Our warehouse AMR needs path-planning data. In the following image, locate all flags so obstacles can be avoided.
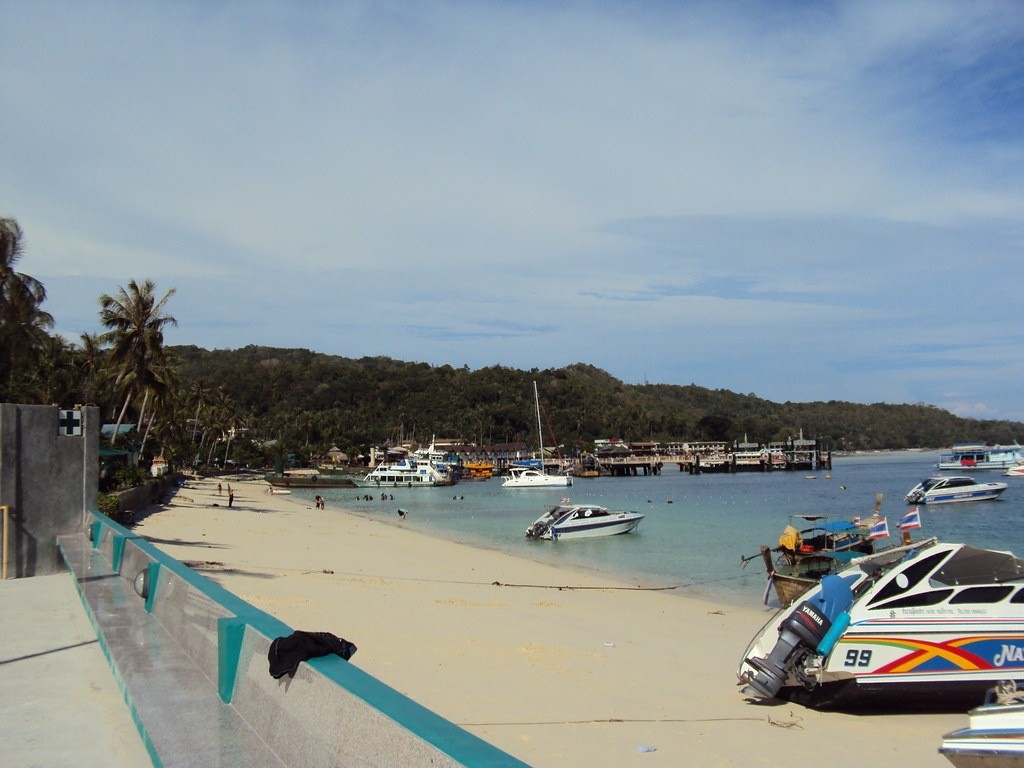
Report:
[897,508,922,530]
[867,517,890,540]
[852,516,861,527]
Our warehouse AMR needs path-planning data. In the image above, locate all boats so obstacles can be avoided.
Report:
[524,504,646,541]
[1001,465,1024,478]
[568,452,599,477]
[903,474,1009,505]
[733,504,1023,768]
[263,473,359,489]
[361,434,495,487]
[936,442,1024,469]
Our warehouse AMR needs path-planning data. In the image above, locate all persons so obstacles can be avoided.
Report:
[216,482,234,507]
[315,495,325,510]
[398,508,409,521]
[355,493,395,501]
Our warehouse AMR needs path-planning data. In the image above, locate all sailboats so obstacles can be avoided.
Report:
[500,381,574,488]
